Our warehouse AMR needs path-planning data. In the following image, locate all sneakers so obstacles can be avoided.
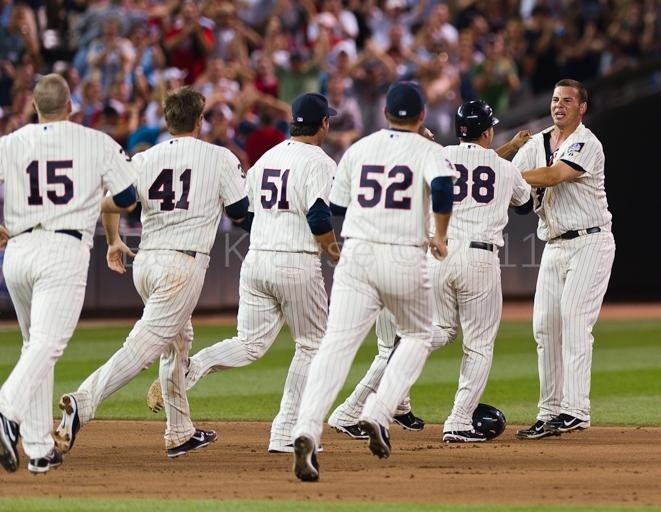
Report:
[328,421,370,439]
[146,358,192,414]
[442,428,487,443]
[268,443,324,453]
[292,433,320,482]
[516,420,553,439]
[28,447,64,476]
[543,413,591,434]
[0,412,20,474]
[52,392,79,456]
[357,417,392,460]
[392,411,426,432]
[167,428,219,459]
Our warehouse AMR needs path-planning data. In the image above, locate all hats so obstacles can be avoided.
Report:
[291,92,338,124]
[455,98,500,138]
[386,80,427,119]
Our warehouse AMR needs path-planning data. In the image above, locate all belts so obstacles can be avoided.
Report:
[175,249,196,258]
[445,239,494,252]
[21,227,82,240]
[555,227,601,240]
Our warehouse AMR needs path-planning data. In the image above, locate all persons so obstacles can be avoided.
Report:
[326,126,532,440]
[51,87,250,458]
[1,0,659,175]
[510,77,617,440]
[144,91,340,457]
[0,72,139,476]
[385,98,541,440]
[292,81,458,485]
